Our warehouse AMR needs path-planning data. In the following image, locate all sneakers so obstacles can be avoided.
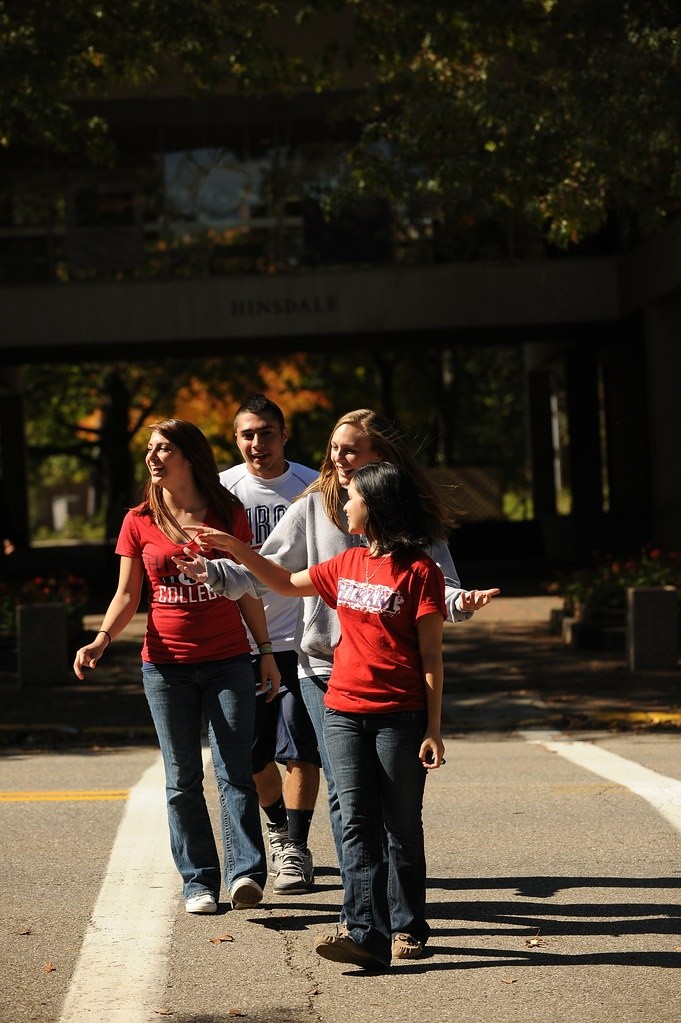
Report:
[185,893,217,913]
[230,878,263,910]
[273,839,314,894]
[391,933,423,957]
[266,822,291,876]
[314,926,389,971]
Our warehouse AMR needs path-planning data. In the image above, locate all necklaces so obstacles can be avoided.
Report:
[365,551,389,587]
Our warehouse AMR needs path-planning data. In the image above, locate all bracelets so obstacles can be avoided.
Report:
[258,641,274,655]
[97,631,111,646]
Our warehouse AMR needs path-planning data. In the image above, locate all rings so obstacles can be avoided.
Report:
[193,574,197,579]
[440,759,446,765]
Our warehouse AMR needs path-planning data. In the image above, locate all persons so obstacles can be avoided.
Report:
[73,416,281,910]
[180,460,464,969]
[171,410,500,936]
[213,395,323,889]
